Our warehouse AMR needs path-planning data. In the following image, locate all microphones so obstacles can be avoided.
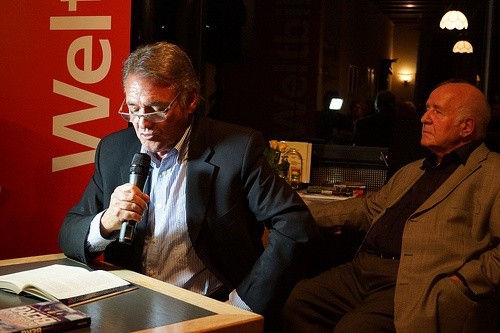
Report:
[118,153,151,245]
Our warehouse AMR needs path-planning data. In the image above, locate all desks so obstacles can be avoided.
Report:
[0,253,264,333]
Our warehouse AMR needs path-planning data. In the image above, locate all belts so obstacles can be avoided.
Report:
[360,245,400,261]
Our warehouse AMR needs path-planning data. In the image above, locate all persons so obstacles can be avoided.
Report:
[57,40,318,333]
[262,82,500,333]
[206,71,472,186]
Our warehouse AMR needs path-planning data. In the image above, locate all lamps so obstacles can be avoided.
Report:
[439,7,469,31]
[452,35,474,54]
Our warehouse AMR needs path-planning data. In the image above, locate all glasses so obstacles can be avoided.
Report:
[117,84,184,123]
